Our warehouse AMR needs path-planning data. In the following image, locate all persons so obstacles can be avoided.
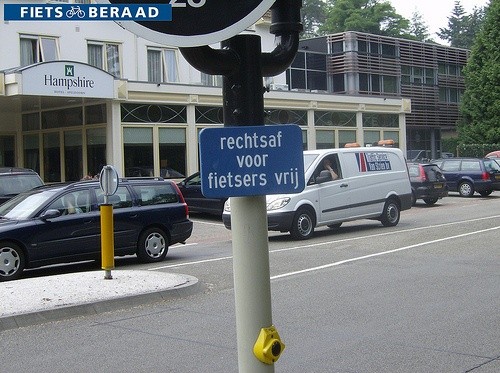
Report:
[324,158,338,180]
[60,194,83,216]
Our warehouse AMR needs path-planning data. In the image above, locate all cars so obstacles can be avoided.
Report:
[177,171,228,218]
[406,146,455,162]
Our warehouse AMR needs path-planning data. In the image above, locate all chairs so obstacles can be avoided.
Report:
[77,193,86,213]
[49,199,62,209]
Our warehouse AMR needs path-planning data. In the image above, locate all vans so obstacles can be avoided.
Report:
[0,176,193,281]
[222,146,413,240]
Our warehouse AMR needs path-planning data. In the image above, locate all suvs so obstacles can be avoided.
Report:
[0,167,46,207]
[430,157,500,197]
[407,162,447,206]
